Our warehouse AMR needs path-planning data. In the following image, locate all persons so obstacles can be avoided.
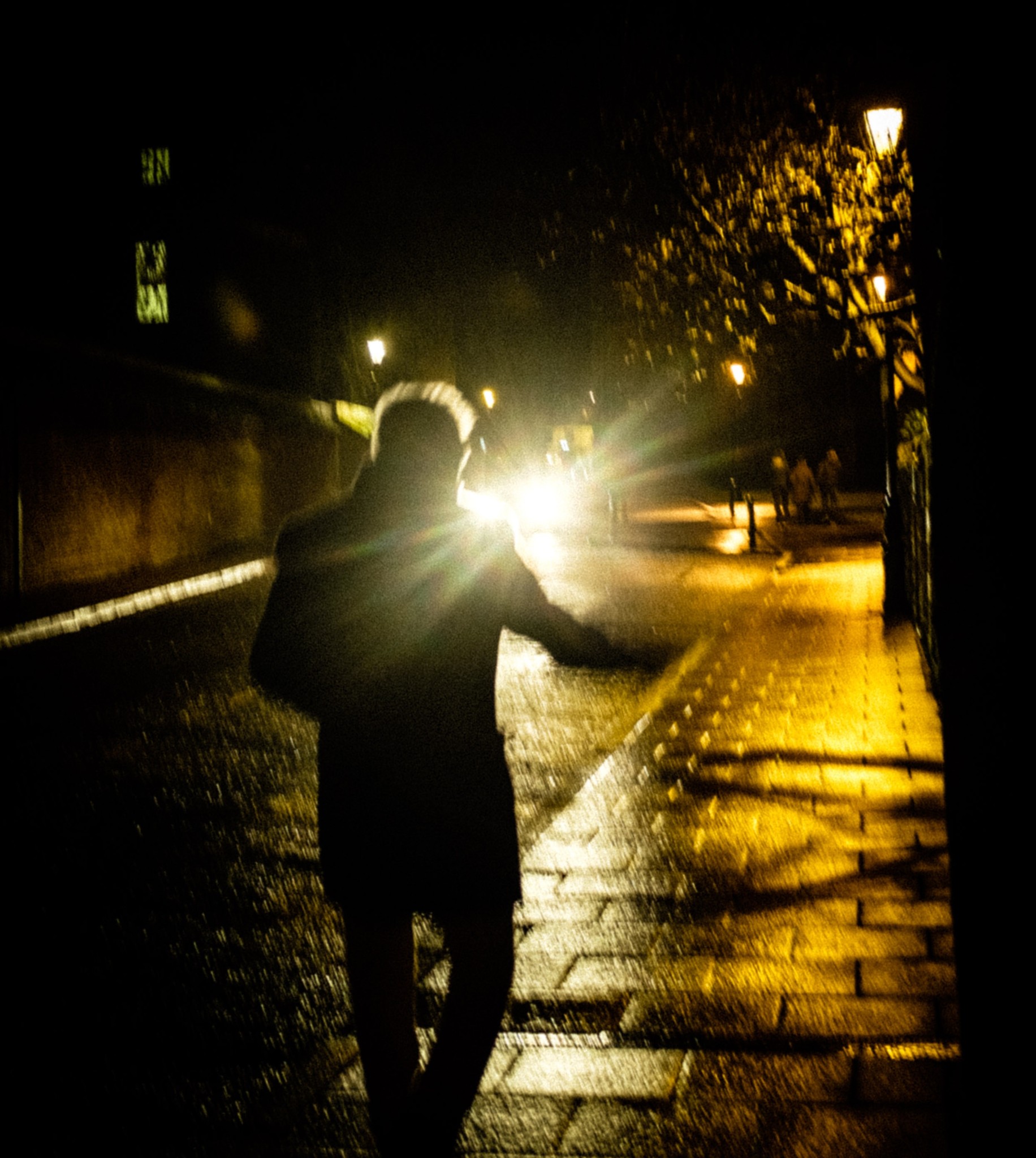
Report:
[249,388,616,1158]
[817,449,842,524]
[789,455,818,522]
[769,449,792,521]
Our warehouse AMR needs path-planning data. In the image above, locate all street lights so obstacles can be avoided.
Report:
[860,104,908,639]
[728,360,758,555]
